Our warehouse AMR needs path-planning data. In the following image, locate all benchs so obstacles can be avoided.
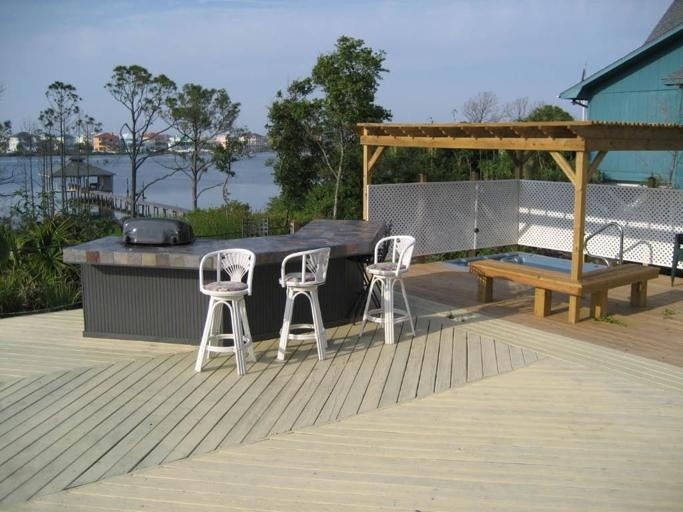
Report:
[468,258,661,324]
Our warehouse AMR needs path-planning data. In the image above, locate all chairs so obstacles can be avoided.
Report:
[358,234,416,345]
[193,247,257,376]
[343,219,391,325]
[275,247,332,361]
